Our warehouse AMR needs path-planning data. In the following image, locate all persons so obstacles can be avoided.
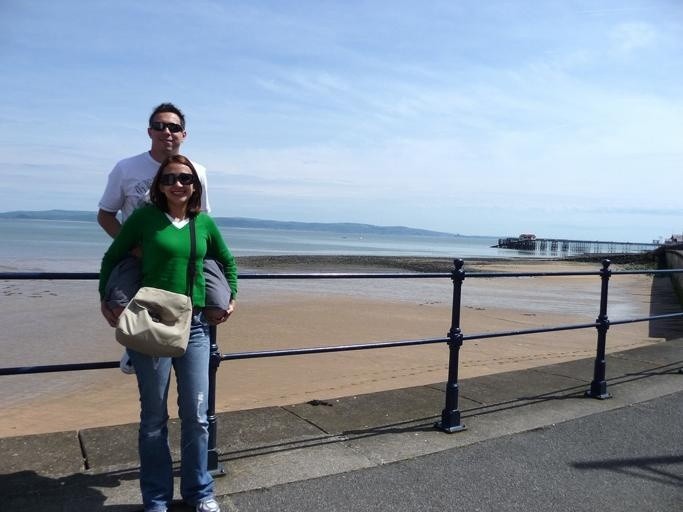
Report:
[98,152,240,511]
[97,102,232,373]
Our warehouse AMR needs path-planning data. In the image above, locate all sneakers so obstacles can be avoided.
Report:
[183,496,221,512]
[120,351,136,374]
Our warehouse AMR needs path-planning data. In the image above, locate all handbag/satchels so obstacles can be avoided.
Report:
[115,287,193,358]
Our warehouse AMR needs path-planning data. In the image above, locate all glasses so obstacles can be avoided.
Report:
[150,122,183,132]
[158,174,195,185]
